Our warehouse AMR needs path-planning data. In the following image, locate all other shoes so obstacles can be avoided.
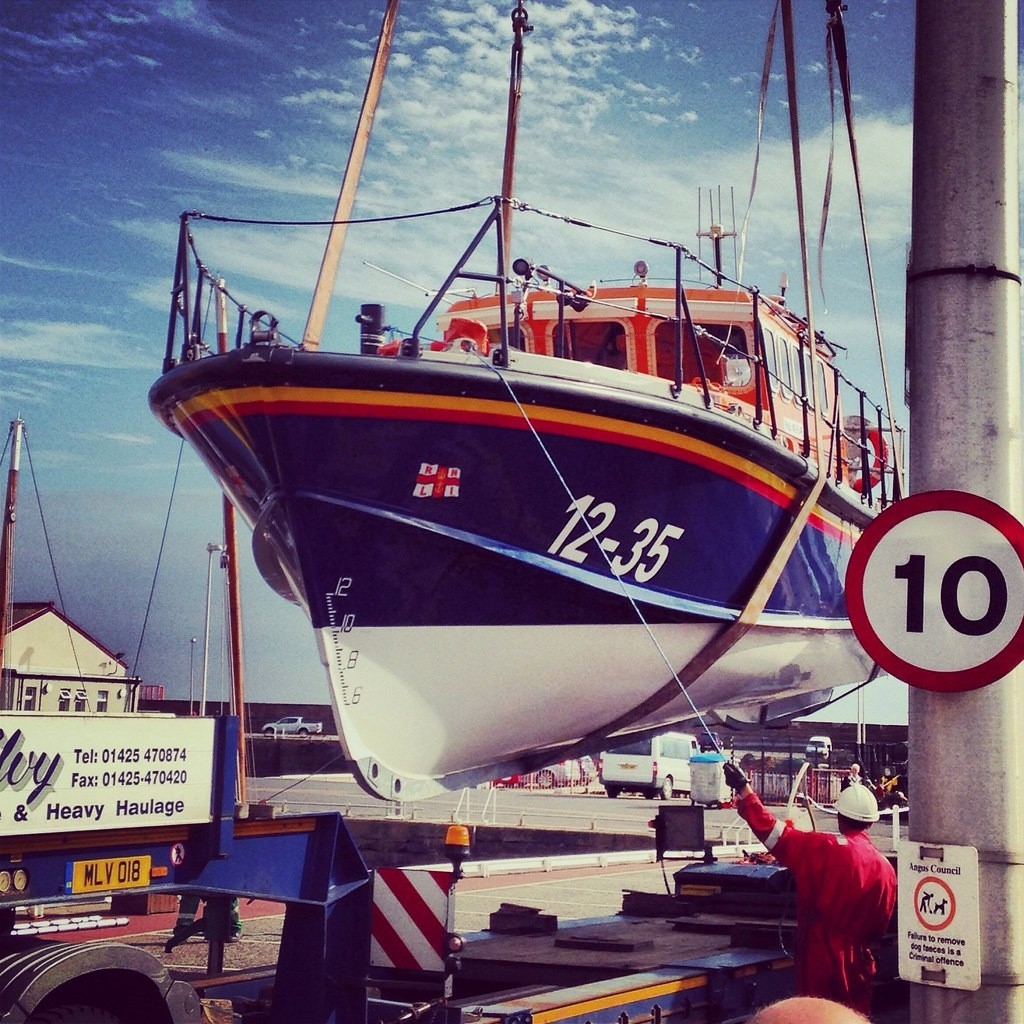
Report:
[226,930,242,942]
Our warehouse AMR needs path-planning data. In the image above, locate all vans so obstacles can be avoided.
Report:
[597,730,701,800]
[805,735,832,760]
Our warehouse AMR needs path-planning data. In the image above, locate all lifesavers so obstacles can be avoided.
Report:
[848,431,890,492]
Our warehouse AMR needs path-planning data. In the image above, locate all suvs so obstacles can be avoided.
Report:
[698,731,725,755]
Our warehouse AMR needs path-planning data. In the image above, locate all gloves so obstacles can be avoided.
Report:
[723,764,752,793]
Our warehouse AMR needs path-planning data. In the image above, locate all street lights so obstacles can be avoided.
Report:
[198,542,224,716]
[189,637,198,716]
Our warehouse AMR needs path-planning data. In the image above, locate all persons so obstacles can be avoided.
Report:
[722,762,897,1019]
[840,764,864,792]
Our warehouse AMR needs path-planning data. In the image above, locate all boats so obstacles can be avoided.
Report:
[140,0,908,806]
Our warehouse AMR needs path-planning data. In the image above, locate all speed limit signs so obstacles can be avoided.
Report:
[843,490,1024,695]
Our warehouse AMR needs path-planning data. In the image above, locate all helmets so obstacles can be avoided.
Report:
[834,783,879,822]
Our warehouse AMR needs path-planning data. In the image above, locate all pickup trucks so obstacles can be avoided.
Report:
[261,716,323,738]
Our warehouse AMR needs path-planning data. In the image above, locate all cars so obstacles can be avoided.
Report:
[492,755,598,790]
